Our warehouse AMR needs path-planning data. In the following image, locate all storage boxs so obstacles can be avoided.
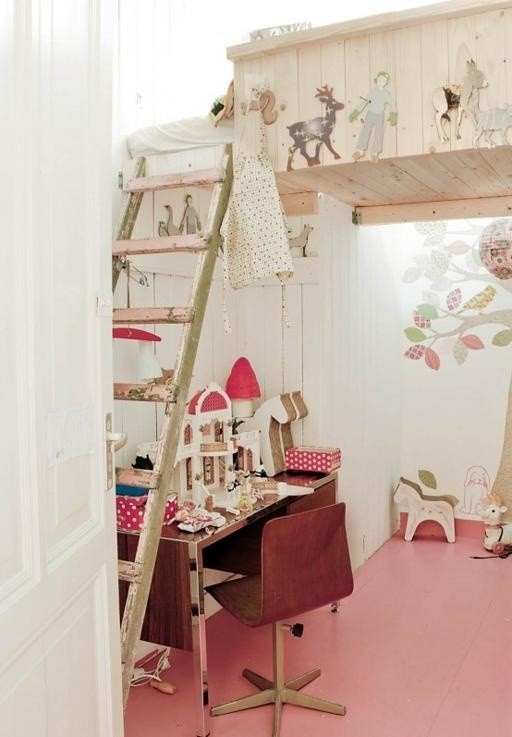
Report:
[117,492,177,530]
[286,445,343,475]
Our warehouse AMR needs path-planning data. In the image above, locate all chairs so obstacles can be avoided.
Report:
[206,503,353,735]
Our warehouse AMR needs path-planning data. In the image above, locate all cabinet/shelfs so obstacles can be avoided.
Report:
[118,453,339,655]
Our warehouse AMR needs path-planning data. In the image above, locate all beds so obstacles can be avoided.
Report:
[124,98,252,159]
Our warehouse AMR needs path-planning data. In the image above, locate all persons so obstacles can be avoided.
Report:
[178,194,204,237]
[346,71,398,164]
